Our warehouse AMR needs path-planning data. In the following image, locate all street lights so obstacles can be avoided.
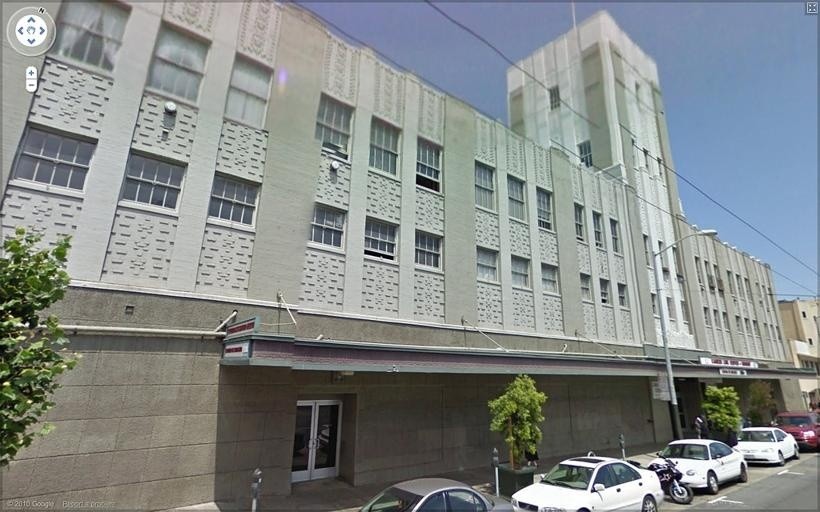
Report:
[650,227,718,440]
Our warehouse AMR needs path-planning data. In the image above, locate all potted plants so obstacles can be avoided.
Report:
[487,371,550,499]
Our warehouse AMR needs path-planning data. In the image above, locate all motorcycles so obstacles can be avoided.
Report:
[625,452,695,505]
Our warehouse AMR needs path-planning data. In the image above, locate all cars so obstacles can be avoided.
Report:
[646,438,748,495]
[510,455,664,512]
[767,410,820,453]
[358,477,514,511]
[734,426,800,469]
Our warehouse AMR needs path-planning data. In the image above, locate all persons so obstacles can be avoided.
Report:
[743,416,752,429]
[690,411,704,440]
[524,448,540,468]
[809,398,820,414]
